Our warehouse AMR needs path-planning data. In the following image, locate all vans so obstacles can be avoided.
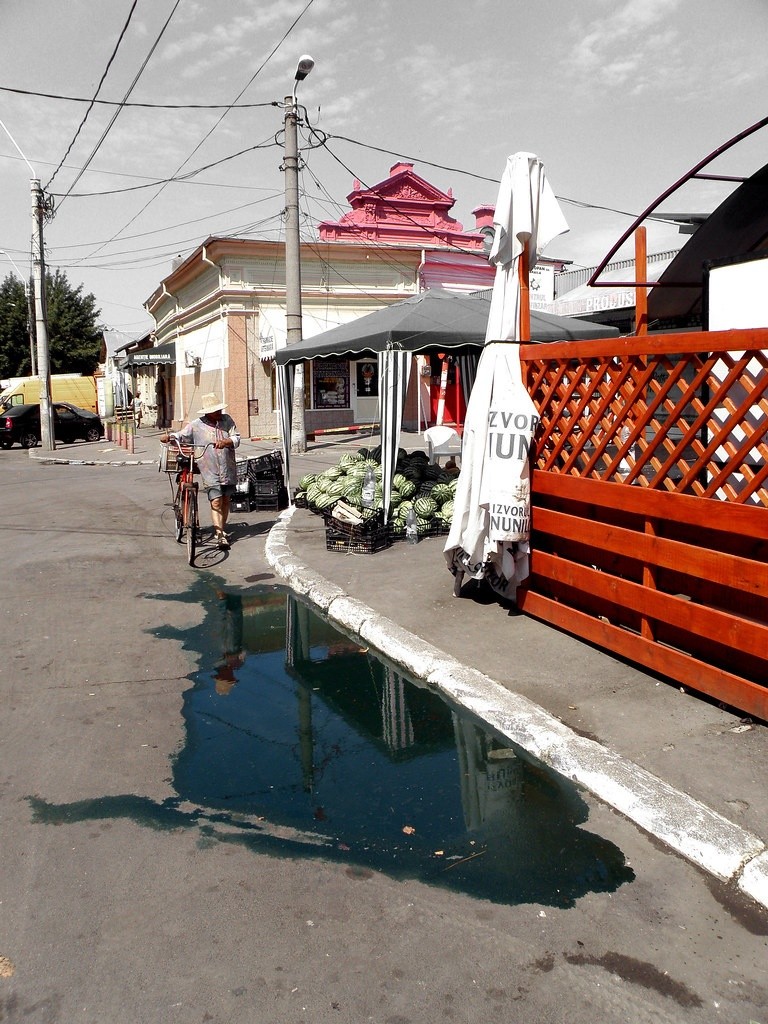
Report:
[0,377,99,416]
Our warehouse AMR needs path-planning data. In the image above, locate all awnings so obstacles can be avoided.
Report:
[117,342,176,371]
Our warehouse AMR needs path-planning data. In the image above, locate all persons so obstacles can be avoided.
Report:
[161,393,240,548]
[130,391,142,428]
[210,591,247,694]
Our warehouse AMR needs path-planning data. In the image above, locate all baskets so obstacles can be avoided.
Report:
[229,450,287,512]
[323,496,385,555]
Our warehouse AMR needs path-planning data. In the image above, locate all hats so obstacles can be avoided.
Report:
[196,392,228,414]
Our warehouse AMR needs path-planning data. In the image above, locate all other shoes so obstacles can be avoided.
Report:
[214,530,231,540]
[215,539,229,549]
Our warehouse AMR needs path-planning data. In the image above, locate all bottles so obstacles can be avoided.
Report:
[362,469,376,508]
[407,507,417,547]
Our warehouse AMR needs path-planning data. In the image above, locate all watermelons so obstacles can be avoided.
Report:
[295,444,462,535]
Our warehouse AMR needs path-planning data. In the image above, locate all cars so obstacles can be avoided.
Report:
[0,402,106,450]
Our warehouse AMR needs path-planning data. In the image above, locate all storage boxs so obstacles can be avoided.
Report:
[230,493,256,513]
[322,497,392,554]
[255,466,283,479]
[250,452,283,472]
[235,476,254,493]
[256,488,288,512]
[254,476,284,496]
[162,446,201,474]
[236,460,254,481]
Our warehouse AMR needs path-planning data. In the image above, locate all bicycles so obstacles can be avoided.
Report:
[171,436,216,567]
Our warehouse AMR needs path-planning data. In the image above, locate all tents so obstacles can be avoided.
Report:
[275,288,620,526]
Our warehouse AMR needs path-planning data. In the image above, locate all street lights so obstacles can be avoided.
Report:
[284,52,317,455]
[0,249,37,376]
